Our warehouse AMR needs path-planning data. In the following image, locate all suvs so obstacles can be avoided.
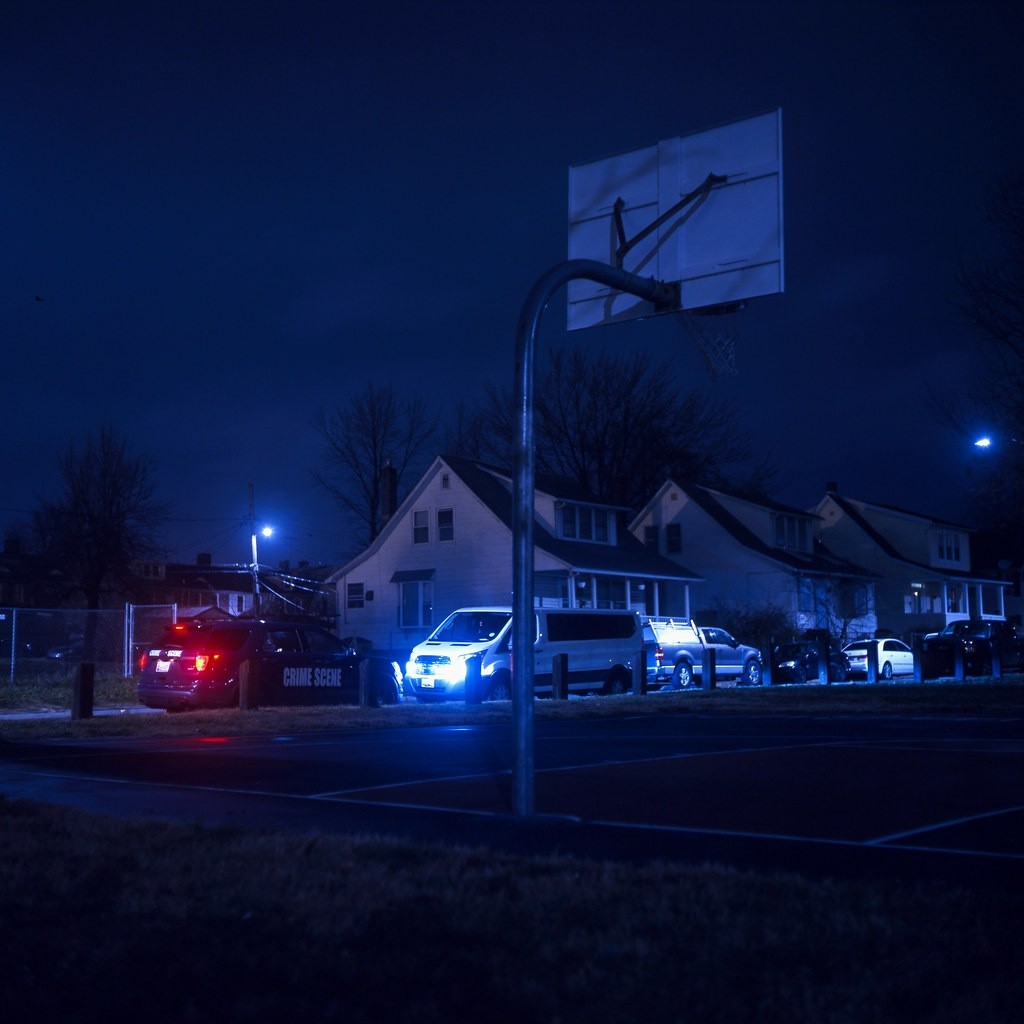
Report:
[136,618,359,709]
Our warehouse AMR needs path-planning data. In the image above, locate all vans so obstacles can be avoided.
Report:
[403,606,648,703]
[640,615,762,689]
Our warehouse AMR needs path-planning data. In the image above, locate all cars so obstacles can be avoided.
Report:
[338,636,398,707]
[773,641,849,685]
[840,639,916,680]
[97,647,121,662]
[916,616,1023,676]
[24,641,47,657]
[45,648,72,662]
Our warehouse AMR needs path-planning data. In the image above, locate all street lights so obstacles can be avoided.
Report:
[252,526,272,611]
